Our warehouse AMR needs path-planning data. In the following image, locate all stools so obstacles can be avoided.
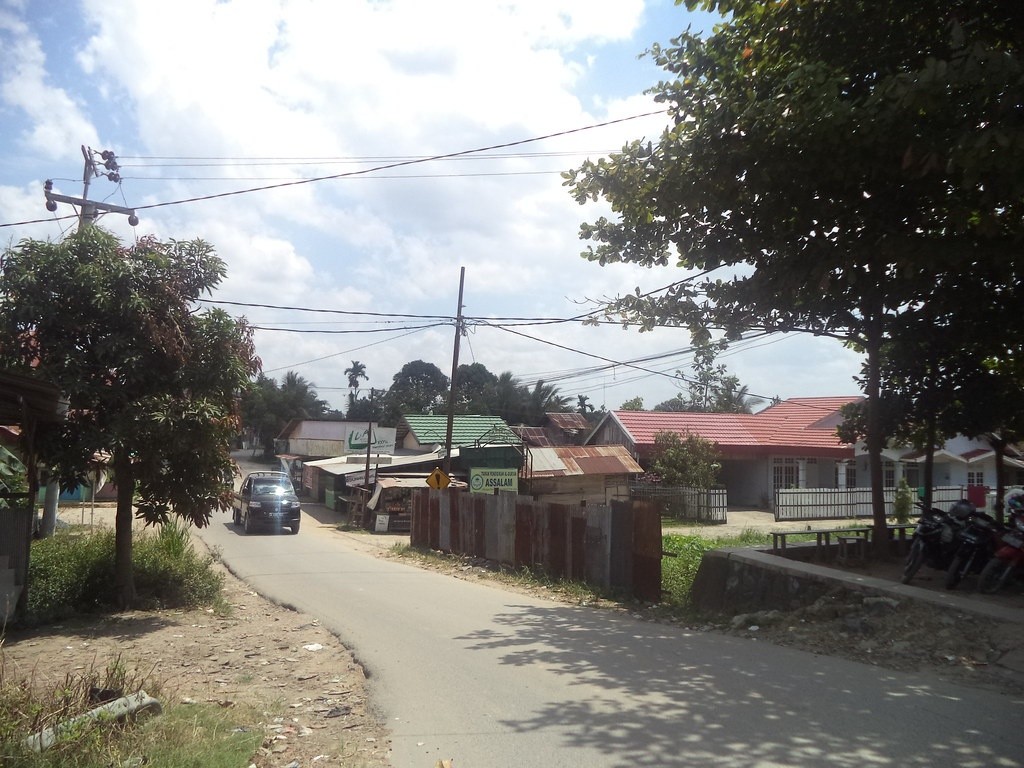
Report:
[836,536,867,570]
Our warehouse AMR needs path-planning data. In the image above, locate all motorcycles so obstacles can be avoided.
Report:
[903,496,977,585]
[944,504,1003,591]
[974,488,1024,595]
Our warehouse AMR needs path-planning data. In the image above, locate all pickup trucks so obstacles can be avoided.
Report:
[230,470,302,535]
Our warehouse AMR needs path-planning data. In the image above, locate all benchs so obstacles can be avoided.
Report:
[867,524,917,556]
[769,527,872,561]
